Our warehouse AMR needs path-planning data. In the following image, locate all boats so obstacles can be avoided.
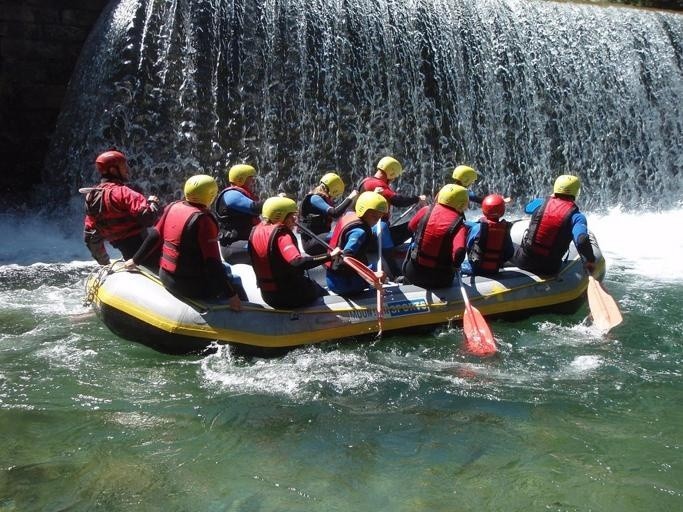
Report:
[83,227,606,356]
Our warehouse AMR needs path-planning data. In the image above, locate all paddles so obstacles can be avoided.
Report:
[295,222,379,287]
[455,269,496,356]
[376,219,385,329]
[572,237,623,330]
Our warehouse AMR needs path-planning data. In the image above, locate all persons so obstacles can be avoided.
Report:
[359,155,426,277]
[453,167,483,228]
[124,175,249,311]
[388,184,470,290]
[214,164,286,265]
[299,172,360,256]
[508,175,596,276]
[78,150,162,275]
[324,191,389,297]
[248,196,344,310]
[461,193,515,279]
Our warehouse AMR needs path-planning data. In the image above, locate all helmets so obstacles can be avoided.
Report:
[452,165,478,186]
[320,173,345,197]
[261,197,300,225]
[354,190,389,218]
[184,174,219,209]
[439,183,469,213]
[229,164,257,187]
[482,194,505,220]
[553,175,581,202]
[377,156,403,180]
[96,152,129,176]
